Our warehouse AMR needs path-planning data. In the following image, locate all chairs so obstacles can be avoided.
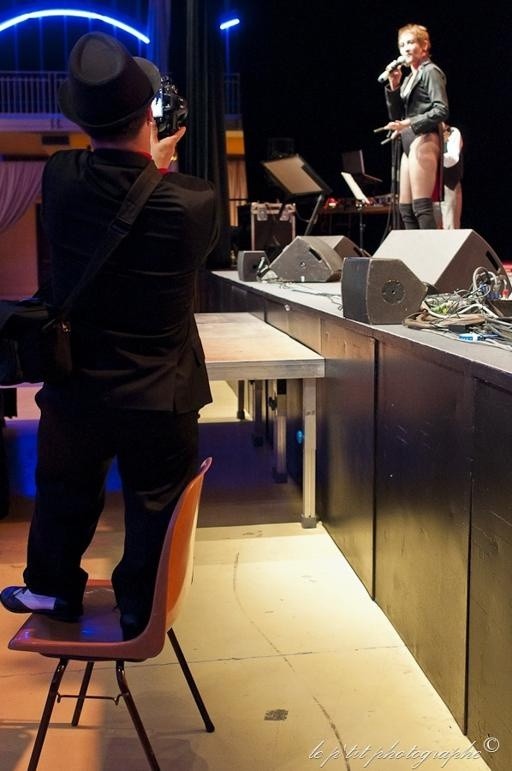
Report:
[8,457,214,771]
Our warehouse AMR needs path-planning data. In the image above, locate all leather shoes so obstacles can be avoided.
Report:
[118,604,148,631]
[0,585,85,622]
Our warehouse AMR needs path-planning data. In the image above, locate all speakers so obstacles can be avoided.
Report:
[237,202,296,264]
[270,236,343,282]
[371,229,512,300]
[318,236,359,261]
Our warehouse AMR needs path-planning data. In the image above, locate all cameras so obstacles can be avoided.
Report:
[151,74,190,140]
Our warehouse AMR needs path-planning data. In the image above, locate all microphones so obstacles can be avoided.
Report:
[378,56,405,84]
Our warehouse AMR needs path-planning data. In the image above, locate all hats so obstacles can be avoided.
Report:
[58,32,162,132]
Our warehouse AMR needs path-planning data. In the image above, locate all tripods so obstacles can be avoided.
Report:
[357,203,370,257]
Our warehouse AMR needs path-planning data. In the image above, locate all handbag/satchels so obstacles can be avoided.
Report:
[1,297,81,388]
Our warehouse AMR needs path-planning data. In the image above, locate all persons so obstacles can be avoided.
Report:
[385,24,450,229]
[0,29,218,640]
[434,122,464,232]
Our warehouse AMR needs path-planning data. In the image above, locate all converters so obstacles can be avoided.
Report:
[449,323,468,334]
